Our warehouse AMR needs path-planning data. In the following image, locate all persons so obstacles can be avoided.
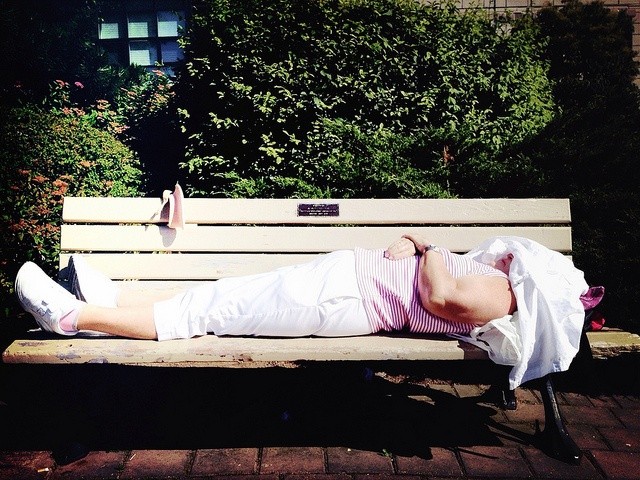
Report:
[13,234,519,342]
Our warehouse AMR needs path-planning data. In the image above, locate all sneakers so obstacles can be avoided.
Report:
[68,255,119,335]
[14,261,79,336]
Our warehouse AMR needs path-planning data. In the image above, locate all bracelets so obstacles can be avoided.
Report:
[422,244,442,255]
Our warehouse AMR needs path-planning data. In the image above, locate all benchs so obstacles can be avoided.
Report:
[3,196,639,462]
